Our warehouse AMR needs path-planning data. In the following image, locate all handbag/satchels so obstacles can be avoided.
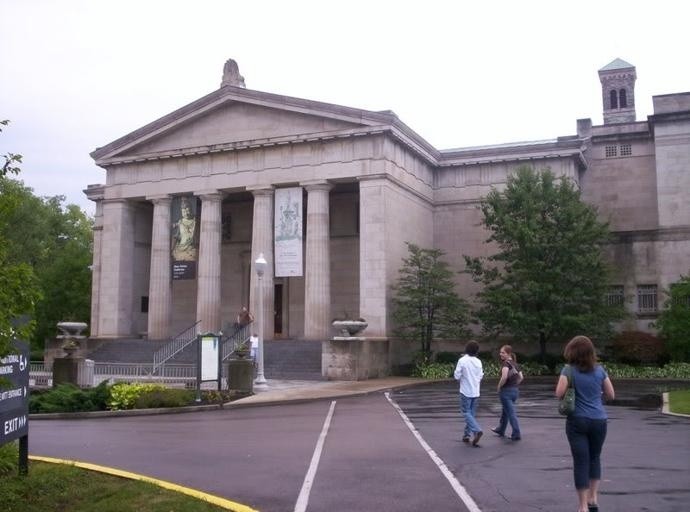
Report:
[506,361,518,384]
[558,366,576,416]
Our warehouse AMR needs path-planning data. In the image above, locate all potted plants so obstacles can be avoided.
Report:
[331,311,371,336]
[55,311,87,336]
[231,344,251,358]
[60,342,81,357]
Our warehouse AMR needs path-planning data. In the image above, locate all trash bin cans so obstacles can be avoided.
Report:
[85,360,94,387]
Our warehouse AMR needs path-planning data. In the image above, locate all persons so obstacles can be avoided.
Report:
[237,306,254,339]
[491,345,525,441]
[555,336,615,512]
[453,340,484,446]
[171,197,198,261]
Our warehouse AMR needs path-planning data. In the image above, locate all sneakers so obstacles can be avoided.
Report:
[472,431,483,445]
[463,438,469,442]
[587,503,599,512]
[491,427,504,436]
[508,436,521,440]
